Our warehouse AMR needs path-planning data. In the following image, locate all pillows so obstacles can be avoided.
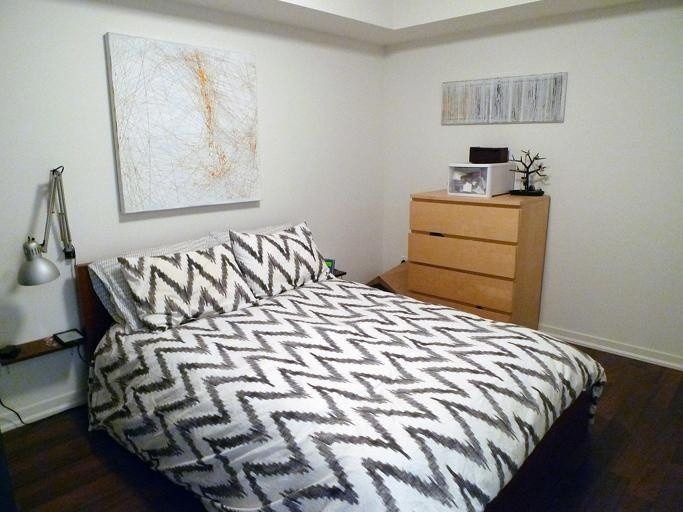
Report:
[87,222,339,329]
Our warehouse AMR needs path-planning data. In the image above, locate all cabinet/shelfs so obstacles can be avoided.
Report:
[405,191,550,331]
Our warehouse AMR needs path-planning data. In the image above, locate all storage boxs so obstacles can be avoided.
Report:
[446,161,515,198]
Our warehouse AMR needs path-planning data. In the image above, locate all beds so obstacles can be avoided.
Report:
[73,221,607,459]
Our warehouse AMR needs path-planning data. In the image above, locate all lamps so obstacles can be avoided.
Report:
[17,166,75,286]
